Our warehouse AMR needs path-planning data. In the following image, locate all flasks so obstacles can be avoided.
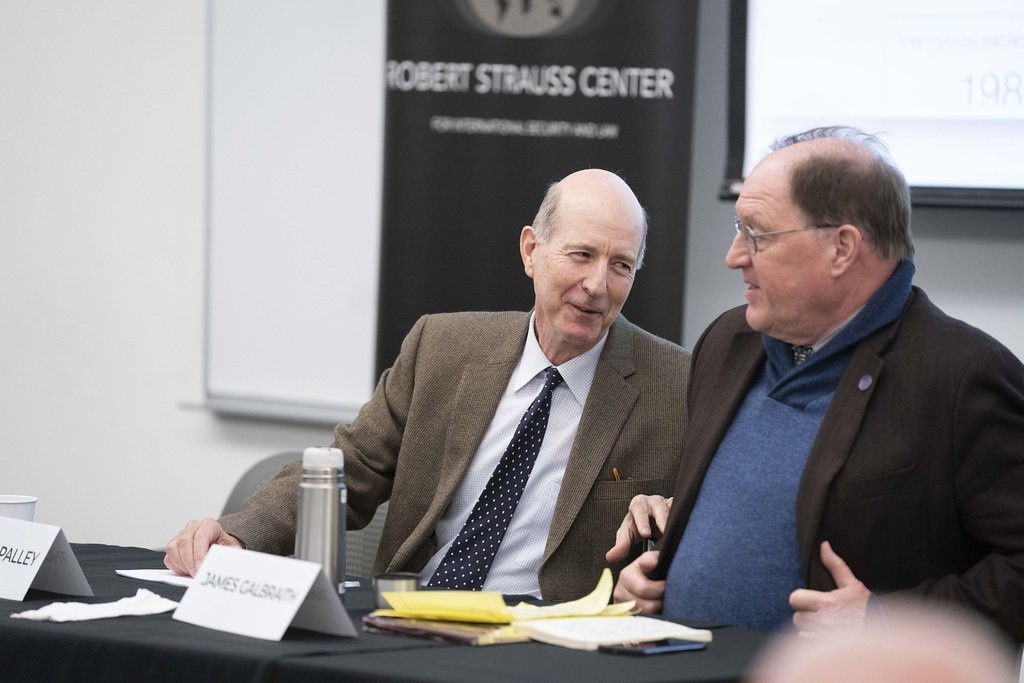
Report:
[293,447,347,609]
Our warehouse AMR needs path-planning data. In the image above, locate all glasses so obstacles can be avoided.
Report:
[733,216,851,256]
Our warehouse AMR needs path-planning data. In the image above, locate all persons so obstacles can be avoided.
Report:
[613,126,1024,648]
[163,170,692,608]
[754,597,1008,683]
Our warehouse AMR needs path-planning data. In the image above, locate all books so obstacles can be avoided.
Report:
[363,568,712,650]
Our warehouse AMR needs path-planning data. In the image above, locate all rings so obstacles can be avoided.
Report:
[666,497,673,505]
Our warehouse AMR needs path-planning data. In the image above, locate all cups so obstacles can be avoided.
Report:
[0,495,38,523]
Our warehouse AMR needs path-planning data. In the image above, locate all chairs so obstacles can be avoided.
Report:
[222,451,391,576]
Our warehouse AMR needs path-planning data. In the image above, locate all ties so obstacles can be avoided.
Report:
[425,366,564,589]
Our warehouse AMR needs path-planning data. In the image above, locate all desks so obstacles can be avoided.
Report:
[0,538,772,683]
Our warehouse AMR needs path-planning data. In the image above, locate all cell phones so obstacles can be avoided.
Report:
[597,636,706,655]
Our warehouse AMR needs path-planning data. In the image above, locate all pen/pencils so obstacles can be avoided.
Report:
[613,467,621,481]
